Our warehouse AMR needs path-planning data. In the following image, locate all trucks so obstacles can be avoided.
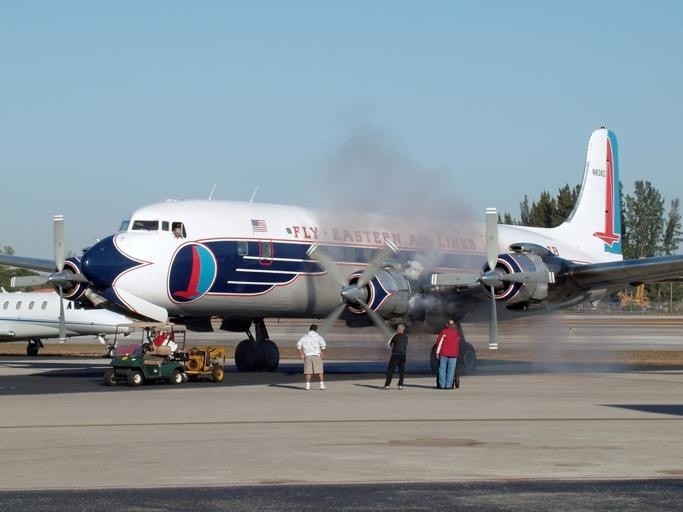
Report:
[108,320,227,386]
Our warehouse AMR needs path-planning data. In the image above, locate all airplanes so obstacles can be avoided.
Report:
[0,284,135,355]
[0,124,683,378]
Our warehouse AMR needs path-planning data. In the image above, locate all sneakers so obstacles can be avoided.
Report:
[397,386,401,390]
[319,385,325,390]
[305,386,310,390]
[381,386,389,390]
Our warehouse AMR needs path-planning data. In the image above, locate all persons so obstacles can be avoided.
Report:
[296,324,327,391]
[436,319,461,389]
[381,324,409,390]
[174,227,182,239]
[152,328,178,358]
